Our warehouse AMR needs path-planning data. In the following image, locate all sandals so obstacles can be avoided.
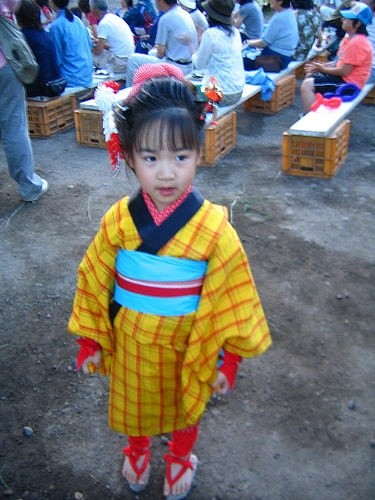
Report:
[123,450,150,492]
[161,455,198,499]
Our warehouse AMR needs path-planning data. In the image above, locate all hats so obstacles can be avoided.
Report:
[178,0,198,11]
[202,0,235,25]
[340,2,372,25]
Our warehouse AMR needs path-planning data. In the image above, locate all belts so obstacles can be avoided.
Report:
[167,57,193,65]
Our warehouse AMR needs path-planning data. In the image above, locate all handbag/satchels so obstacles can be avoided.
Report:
[0,17,39,85]
[45,77,66,97]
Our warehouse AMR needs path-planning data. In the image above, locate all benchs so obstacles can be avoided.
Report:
[289,82,375,137]
[312,37,338,56]
[28,72,126,102]
[205,50,319,128]
[79,86,132,111]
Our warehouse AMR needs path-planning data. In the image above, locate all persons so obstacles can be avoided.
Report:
[125,0,199,88]
[115,0,165,55]
[67,0,135,74]
[14,0,67,98]
[67,63,273,500]
[177,0,209,46]
[0,0,48,201]
[298,4,373,118]
[34,0,93,88]
[191,0,246,108]
[242,0,299,74]
[231,0,264,44]
[290,0,375,84]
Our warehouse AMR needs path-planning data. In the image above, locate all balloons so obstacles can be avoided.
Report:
[310,92,342,111]
[324,83,360,102]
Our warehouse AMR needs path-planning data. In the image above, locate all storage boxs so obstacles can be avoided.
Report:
[292,49,330,81]
[26,94,78,140]
[241,74,296,117]
[74,108,108,149]
[196,111,238,168]
[282,118,351,179]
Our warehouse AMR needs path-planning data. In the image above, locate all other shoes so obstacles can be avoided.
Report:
[25,179,47,203]
